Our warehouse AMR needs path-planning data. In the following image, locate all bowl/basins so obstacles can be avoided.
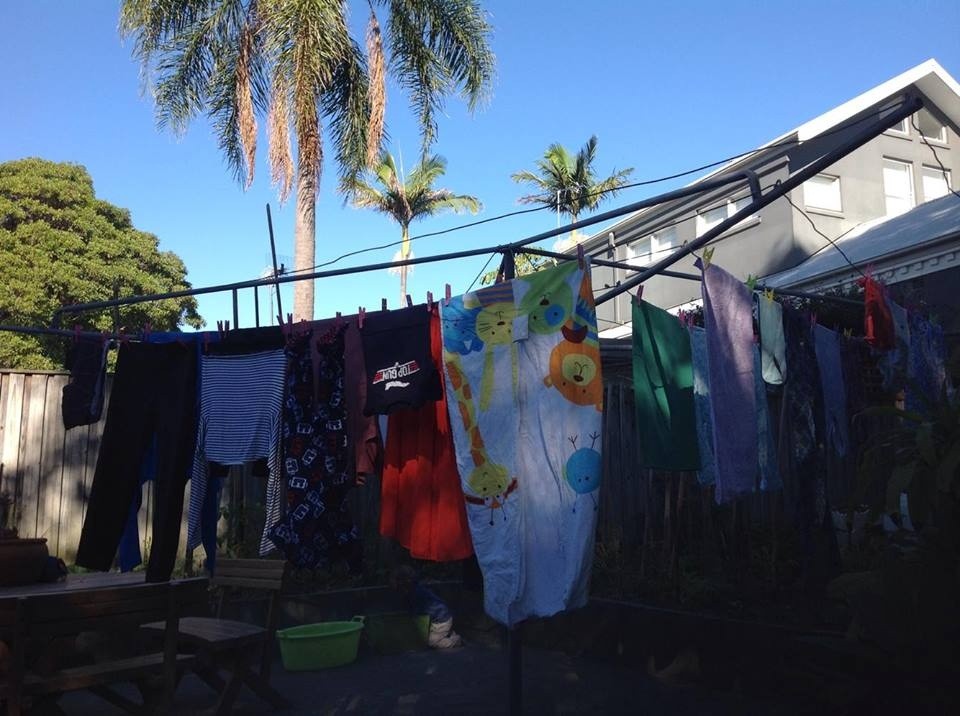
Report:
[275,616,366,670]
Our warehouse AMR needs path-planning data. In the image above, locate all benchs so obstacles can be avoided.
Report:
[0,559,289,716]
